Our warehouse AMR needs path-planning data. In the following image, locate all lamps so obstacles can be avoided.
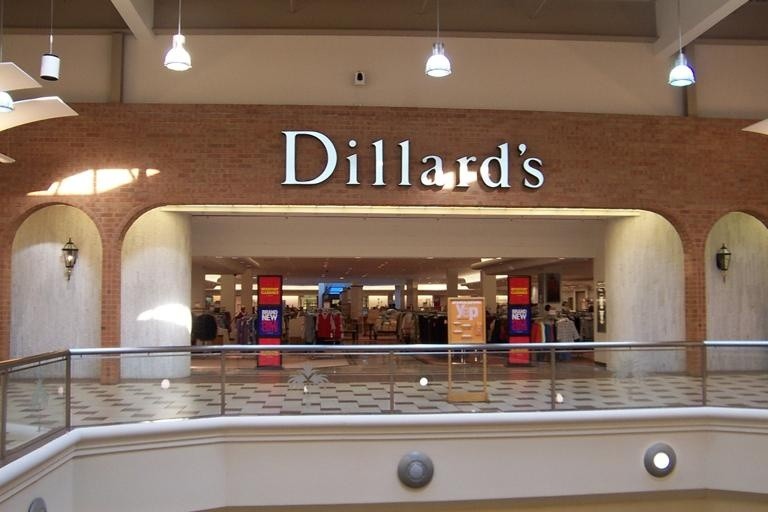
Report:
[0,91,14,112]
[716,241,732,277]
[668,0,696,88]
[165,0,194,72]
[39,0,60,82]
[425,0,451,77]
[62,238,78,280]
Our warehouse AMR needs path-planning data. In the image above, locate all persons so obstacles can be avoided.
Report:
[584,298,594,312]
[485,308,498,342]
[235,304,248,319]
[560,301,571,317]
[545,303,561,316]
[365,306,380,341]
